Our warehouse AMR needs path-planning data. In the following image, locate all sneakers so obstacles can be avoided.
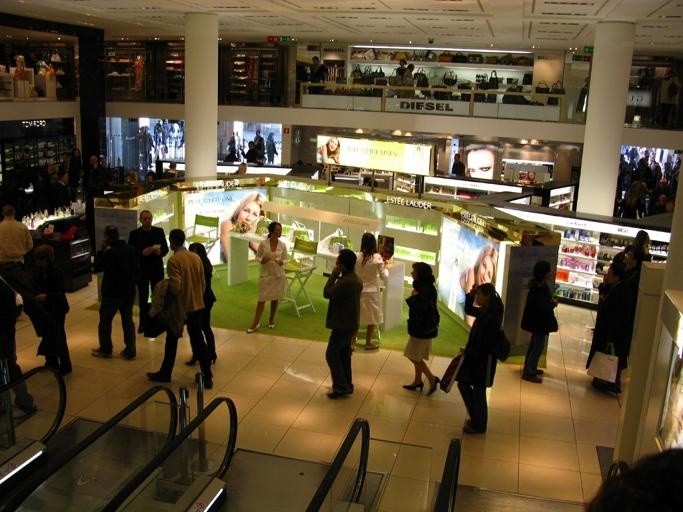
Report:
[92,348,136,360]
[522,369,543,383]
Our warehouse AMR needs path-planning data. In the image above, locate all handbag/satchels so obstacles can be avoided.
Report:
[336,65,428,87]
[536,80,564,105]
[432,70,533,103]
[440,355,463,393]
[352,48,534,66]
[587,351,621,381]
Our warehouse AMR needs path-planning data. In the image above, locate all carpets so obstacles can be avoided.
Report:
[83,260,550,372]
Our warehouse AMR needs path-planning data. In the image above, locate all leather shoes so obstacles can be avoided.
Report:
[248,319,274,333]
[147,372,171,382]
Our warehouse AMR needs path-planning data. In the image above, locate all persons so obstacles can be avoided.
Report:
[89,226,139,359]
[184,243,216,366]
[396,59,405,76]
[451,154,466,179]
[146,229,214,389]
[220,192,267,263]
[308,56,329,93]
[43,148,106,204]
[84,170,157,251]
[613,149,680,224]
[137,120,185,179]
[228,129,278,174]
[469,146,506,185]
[520,260,561,384]
[247,222,288,333]
[128,211,169,334]
[583,230,653,397]
[317,139,339,168]
[308,231,504,434]
[2,205,74,412]
[405,65,414,77]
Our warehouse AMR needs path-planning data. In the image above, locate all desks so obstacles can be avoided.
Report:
[225,229,406,334]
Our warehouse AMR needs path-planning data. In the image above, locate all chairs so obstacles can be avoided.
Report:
[278,237,318,318]
[179,214,218,276]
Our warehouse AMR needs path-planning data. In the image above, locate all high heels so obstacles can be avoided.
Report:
[186,353,217,365]
[403,377,440,395]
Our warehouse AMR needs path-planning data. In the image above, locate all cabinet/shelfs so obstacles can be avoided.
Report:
[345,43,533,90]
[551,212,671,311]
[0,133,77,184]
[105,41,296,110]
